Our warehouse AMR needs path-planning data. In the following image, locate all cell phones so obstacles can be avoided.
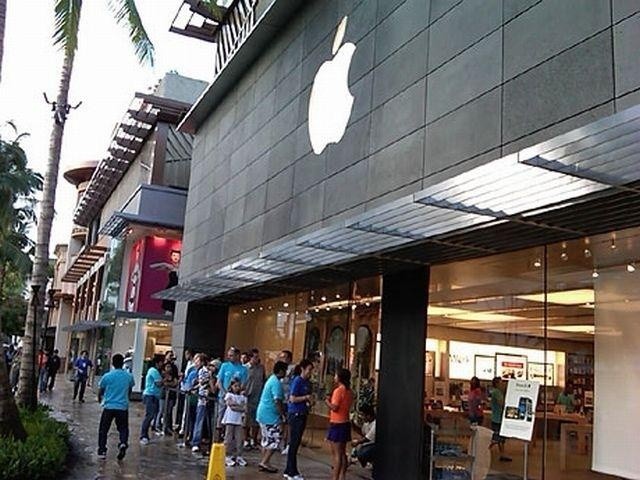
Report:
[519,398,526,419]
[526,398,532,421]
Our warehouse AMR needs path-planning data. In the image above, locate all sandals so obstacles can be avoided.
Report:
[259,461,278,473]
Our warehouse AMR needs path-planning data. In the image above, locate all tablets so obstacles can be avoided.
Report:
[505,406,520,419]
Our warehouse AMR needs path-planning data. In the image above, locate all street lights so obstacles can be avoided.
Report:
[29,282,60,411]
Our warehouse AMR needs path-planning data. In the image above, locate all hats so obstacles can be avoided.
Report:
[207,359,222,369]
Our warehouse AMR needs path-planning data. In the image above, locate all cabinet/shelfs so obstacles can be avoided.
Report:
[555,347,594,407]
[424,423,477,480]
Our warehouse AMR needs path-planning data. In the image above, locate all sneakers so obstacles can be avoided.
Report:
[283,471,305,480]
[116,444,128,460]
[498,454,513,462]
[138,428,199,452]
[233,456,248,466]
[244,439,259,451]
[225,455,236,466]
[95,449,107,459]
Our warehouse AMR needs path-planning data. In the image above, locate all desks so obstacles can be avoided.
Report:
[559,419,595,474]
[531,411,589,454]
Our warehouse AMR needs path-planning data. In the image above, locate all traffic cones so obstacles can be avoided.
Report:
[205,440,226,479]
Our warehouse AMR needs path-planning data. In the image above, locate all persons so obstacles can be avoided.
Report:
[149,249,180,316]
[488,376,513,464]
[469,375,486,427]
[557,383,575,414]
